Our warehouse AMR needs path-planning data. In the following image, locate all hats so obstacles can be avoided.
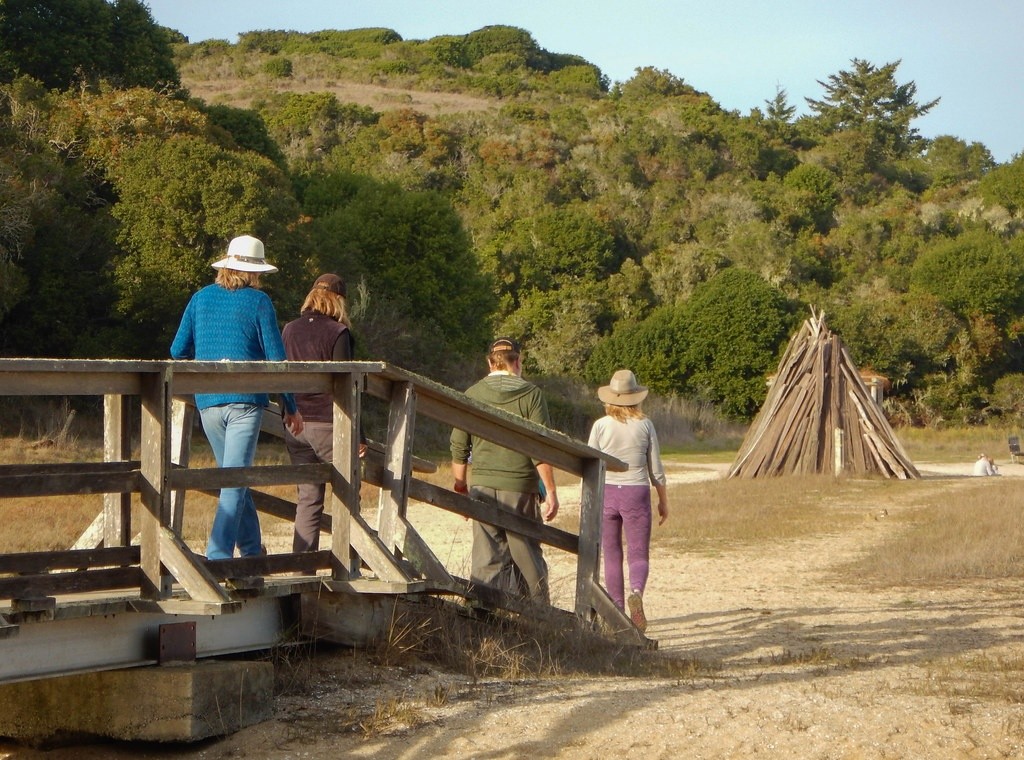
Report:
[598,370,649,407]
[312,273,347,300]
[211,235,279,275]
[488,337,521,355]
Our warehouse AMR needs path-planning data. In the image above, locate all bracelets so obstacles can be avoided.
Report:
[454,484,467,492]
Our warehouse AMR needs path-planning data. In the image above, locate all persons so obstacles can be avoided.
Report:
[281,273,368,576]
[170,235,304,560]
[588,370,669,634]
[974,453,998,476]
[450,337,559,606]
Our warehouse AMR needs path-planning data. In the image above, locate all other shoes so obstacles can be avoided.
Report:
[626,591,648,634]
